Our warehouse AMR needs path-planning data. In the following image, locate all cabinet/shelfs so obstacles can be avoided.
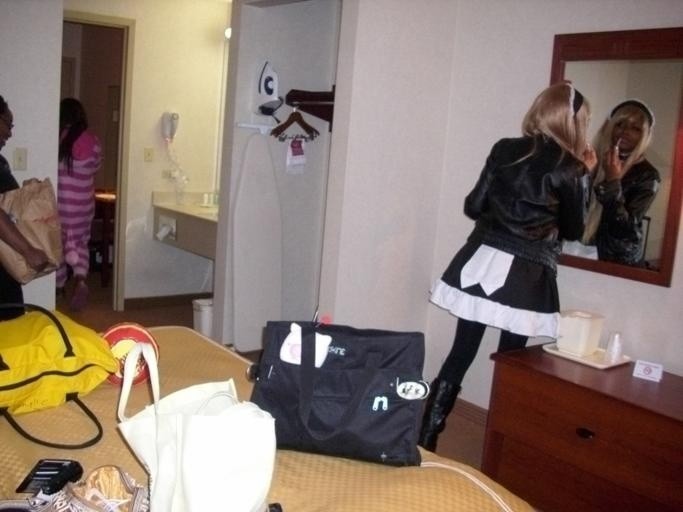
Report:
[480,340,683,510]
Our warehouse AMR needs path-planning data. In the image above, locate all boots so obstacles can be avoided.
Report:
[418,378,461,453]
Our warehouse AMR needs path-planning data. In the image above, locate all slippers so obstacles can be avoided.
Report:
[70,282,88,310]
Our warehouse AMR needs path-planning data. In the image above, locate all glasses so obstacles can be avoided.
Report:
[1,119,17,132]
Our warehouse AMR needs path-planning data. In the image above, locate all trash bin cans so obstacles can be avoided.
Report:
[192,298,212,339]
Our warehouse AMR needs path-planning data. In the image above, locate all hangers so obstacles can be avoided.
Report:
[269,102,319,142]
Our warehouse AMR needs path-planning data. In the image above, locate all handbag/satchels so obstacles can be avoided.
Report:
[0,309,116,414]
[0,177,64,285]
[117,378,277,512]
[247,319,425,468]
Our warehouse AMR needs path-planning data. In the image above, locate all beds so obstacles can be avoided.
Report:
[1,325,534,511]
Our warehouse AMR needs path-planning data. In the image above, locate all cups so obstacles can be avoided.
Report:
[606,330,623,365]
[200,193,216,206]
[555,308,605,358]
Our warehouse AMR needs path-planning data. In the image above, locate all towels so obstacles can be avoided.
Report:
[286,137,307,175]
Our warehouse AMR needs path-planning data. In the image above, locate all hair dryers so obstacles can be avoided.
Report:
[162,112,178,144]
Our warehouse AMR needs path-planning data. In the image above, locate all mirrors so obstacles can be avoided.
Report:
[549,28,683,289]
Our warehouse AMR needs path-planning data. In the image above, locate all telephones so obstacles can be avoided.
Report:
[163,112,179,141]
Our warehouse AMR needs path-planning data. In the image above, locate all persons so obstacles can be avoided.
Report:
[56,98,104,311]
[581,99,662,267]
[0,95,48,320]
[417,79,598,453]
